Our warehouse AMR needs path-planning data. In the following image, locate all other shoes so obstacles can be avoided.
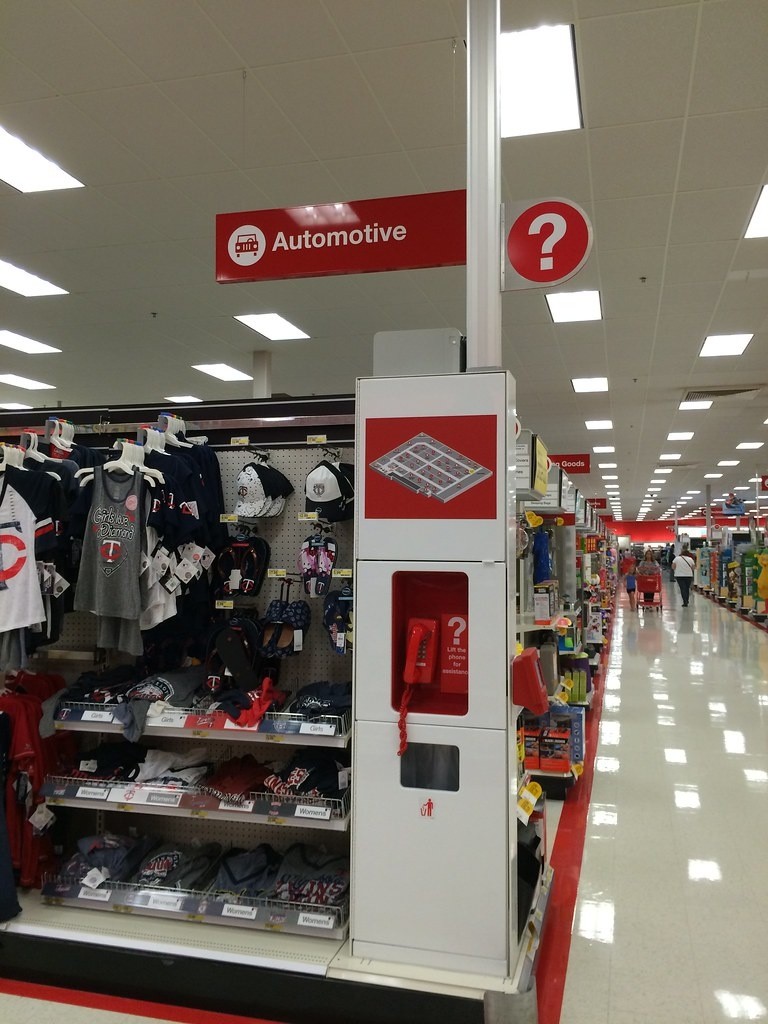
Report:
[633,608,636,612]
[646,607,649,609]
[670,580,676,582]
[631,609,633,611]
[687,600,689,604]
[650,606,652,608]
[256,600,312,659]
[682,604,688,607]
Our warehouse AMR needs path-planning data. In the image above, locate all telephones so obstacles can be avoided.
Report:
[402,617,439,684]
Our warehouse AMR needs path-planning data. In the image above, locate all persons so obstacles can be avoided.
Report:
[619,548,630,560]
[671,551,696,607]
[622,564,637,612]
[638,549,662,613]
[669,547,677,582]
[679,546,697,591]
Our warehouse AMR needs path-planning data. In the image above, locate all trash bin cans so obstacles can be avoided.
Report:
[349,720,518,978]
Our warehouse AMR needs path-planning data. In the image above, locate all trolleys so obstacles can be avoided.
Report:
[635,568,664,613]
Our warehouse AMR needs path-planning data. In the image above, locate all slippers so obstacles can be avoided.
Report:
[217,536,241,596]
[346,600,353,648]
[322,590,345,651]
[298,536,321,594]
[315,536,338,596]
[240,537,271,597]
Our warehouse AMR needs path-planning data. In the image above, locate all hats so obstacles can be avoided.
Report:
[305,460,354,522]
[234,462,295,517]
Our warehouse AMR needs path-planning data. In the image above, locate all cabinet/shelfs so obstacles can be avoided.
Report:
[515,430,617,801]
[40,680,350,940]
